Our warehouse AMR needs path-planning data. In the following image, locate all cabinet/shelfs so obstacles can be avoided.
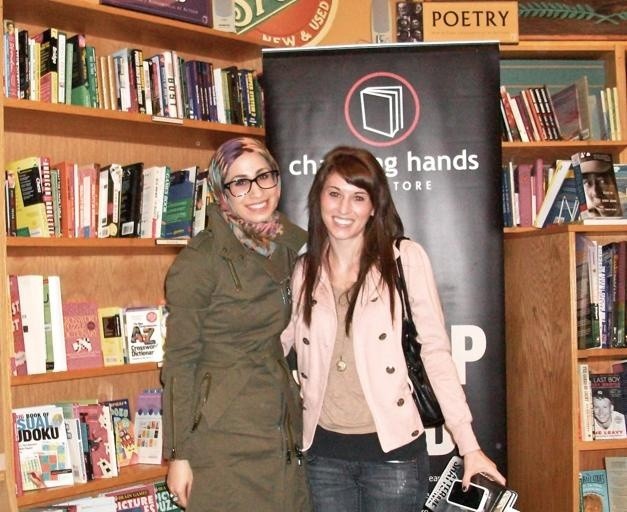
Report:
[493,34,627,512]
[1,2,278,512]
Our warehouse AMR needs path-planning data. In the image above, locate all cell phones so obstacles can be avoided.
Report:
[446,480,490,512]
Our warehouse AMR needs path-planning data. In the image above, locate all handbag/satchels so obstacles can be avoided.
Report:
[401,320,444,430]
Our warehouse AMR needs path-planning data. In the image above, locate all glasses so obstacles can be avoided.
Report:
[221,168,279,198]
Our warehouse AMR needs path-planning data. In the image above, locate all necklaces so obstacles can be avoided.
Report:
[336,331,346,372]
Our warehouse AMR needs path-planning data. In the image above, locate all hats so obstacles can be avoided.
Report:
[578,152,611,175]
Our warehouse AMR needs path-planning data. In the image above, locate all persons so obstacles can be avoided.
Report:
[281,147,519,511]
[157,134,313,511]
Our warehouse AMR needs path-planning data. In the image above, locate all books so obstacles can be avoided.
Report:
[13,388,164,494]
[3,19,261,128]
[574,232,627,350]
[5,156,213,238]
[6,274,168,374]
[503,487,519,512]
[502,77,620,142]
[578,362,627,441]
[31,480,184,512]
[579,457,627,512]
[425,454,512,512]
[501,152,627,228]
